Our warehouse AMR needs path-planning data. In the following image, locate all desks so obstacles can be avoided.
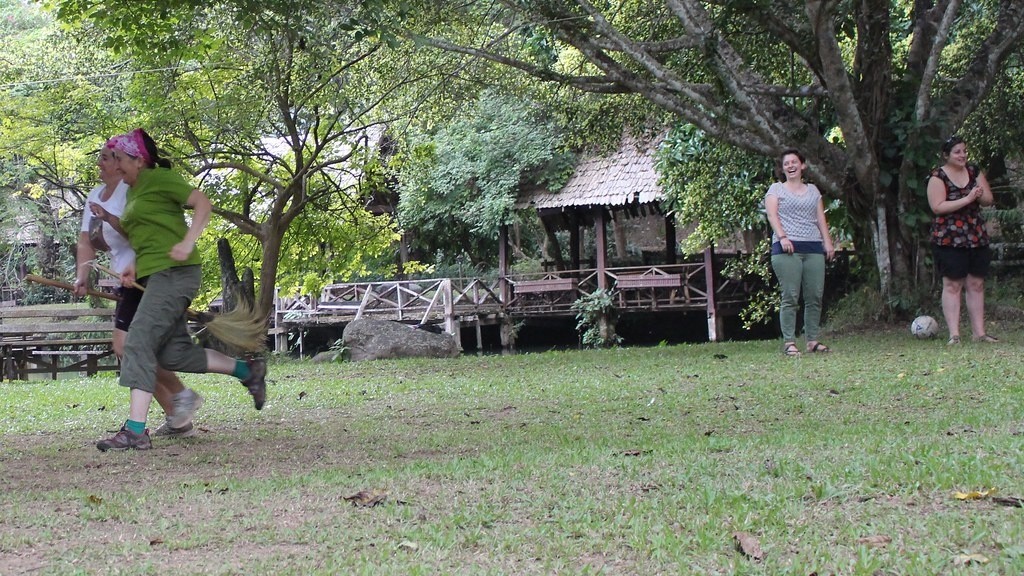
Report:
[616,271,657,307]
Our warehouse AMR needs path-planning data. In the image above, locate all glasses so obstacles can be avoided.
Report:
[943,135,963,152]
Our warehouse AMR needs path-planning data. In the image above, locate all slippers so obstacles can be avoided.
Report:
[806,341,832,353]
[783,344,802,355]
[947,336,962,347]
[971,333,1000,343]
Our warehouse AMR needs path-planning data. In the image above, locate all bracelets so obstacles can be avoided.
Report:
[778,235,787,241]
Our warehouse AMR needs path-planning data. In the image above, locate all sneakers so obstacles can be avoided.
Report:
[152,421,196,440]
[96,419,153,453]
[238,356,267,410]
[169,388,204,429]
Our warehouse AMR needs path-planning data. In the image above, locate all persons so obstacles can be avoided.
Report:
[926,136,1001,347]
[95,127,268,453]
[763,148,836,358]
[71,133,204,441]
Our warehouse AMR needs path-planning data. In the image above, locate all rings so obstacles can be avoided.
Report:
[75,280,81,285]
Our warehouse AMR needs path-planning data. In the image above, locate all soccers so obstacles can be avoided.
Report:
[910,315,940,340]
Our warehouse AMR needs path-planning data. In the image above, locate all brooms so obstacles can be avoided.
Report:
[24,259,276,357]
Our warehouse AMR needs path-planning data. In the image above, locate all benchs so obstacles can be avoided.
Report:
[97,279,120,292]
